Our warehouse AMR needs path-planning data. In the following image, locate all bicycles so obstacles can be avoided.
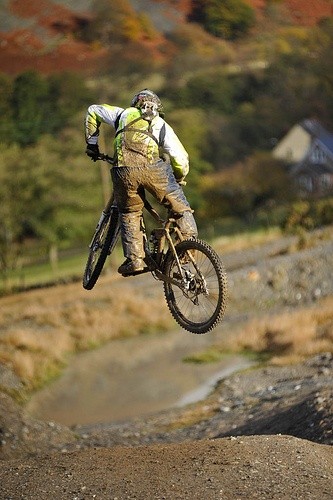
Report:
[81,149,228,335]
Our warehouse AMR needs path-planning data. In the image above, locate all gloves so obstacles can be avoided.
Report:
[86,144,100,162]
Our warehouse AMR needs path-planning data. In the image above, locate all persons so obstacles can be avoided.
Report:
[85,89,198,275]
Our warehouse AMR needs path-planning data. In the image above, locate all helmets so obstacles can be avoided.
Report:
[130,88,163,112]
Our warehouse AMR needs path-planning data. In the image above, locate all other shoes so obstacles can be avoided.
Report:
[176,236,195,264]
[118,258,147,272]
